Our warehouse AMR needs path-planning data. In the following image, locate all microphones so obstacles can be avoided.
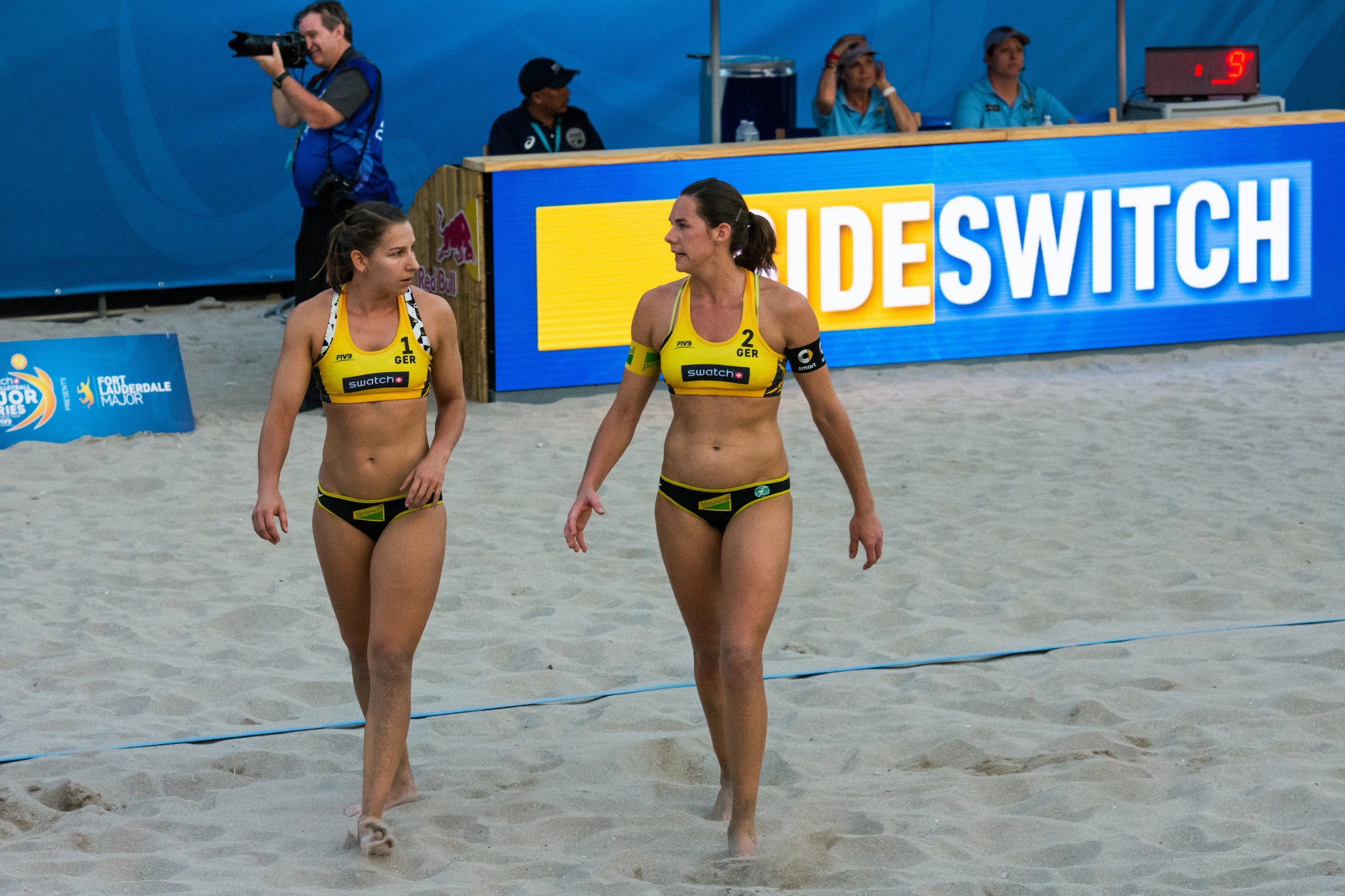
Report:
[1022,67,1026,70]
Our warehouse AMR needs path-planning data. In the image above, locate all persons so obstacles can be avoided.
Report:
[250,0,390,413]
[951,25,1082,131]
[564,178,883,857]
[252,201,466,855]
[488,58,606,157]
[811,34,919,136]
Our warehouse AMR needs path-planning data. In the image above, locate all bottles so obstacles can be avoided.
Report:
[744,121,759,141]
[1042,115,1053,125]
[735,120,748,141]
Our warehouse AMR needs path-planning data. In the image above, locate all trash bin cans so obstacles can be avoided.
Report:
[701,55,797,145]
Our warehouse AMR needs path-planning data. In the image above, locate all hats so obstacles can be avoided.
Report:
[984,25,1031,53]
[518,58,580,95]
[839,39,876,67]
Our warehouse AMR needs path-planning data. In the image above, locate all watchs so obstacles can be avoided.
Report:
[272,70,290,89]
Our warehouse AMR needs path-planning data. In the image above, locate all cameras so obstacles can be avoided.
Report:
[312,172,359,221]
[228,30,309,70]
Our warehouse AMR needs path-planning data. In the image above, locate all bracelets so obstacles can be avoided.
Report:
[880,86,897,98]
[822,52,839,69]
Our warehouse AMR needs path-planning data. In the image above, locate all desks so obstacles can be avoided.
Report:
[405,107,1345,398]
